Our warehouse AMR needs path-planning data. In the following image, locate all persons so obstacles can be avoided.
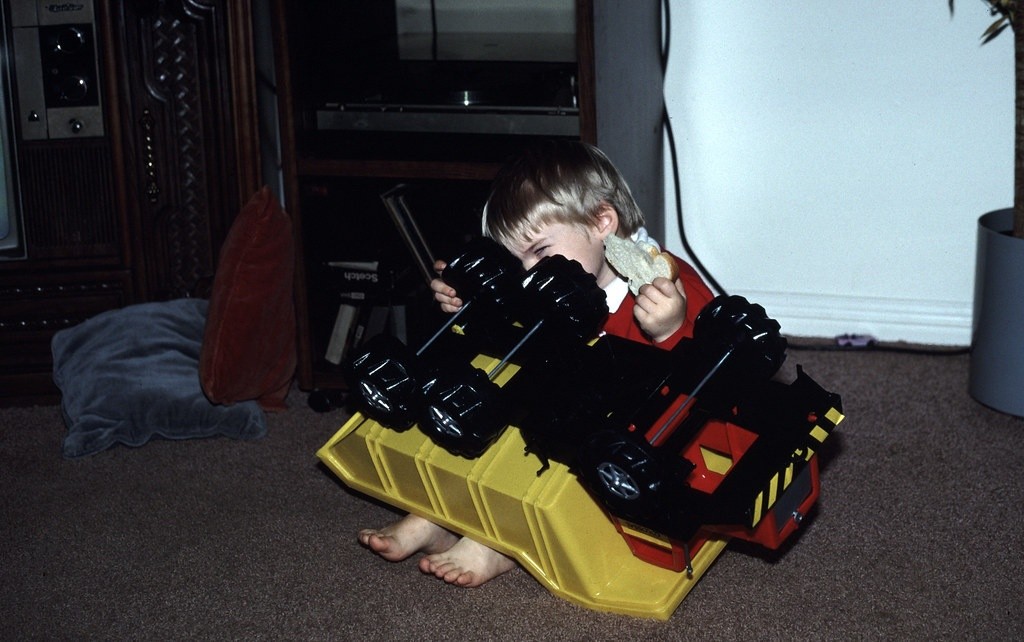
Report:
[357,134,716,589]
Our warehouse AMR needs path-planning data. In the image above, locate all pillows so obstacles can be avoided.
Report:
[51,299,265,460]
[199,184,298,408]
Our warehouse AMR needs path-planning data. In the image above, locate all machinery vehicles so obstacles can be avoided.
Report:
[313,233,846,621]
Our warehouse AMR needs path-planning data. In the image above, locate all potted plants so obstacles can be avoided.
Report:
[950,0,1024,417]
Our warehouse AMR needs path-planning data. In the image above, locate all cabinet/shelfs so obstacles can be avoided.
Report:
[0,0,667,408]
